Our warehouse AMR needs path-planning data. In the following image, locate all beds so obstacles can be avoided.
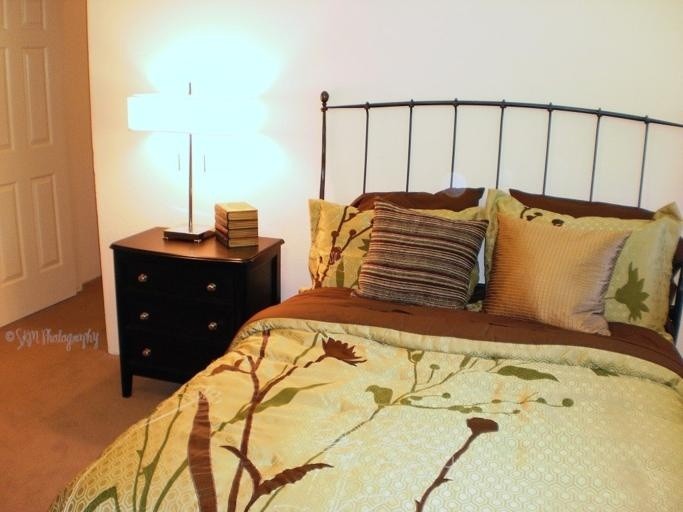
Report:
[45,88,682,510]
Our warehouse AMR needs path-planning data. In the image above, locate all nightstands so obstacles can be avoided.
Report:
[106,225,283,398]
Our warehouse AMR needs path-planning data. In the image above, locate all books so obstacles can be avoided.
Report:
[215,220,259,238]
[215,229,259,249]
[215,213,259,229]
[214,202,258,220]
